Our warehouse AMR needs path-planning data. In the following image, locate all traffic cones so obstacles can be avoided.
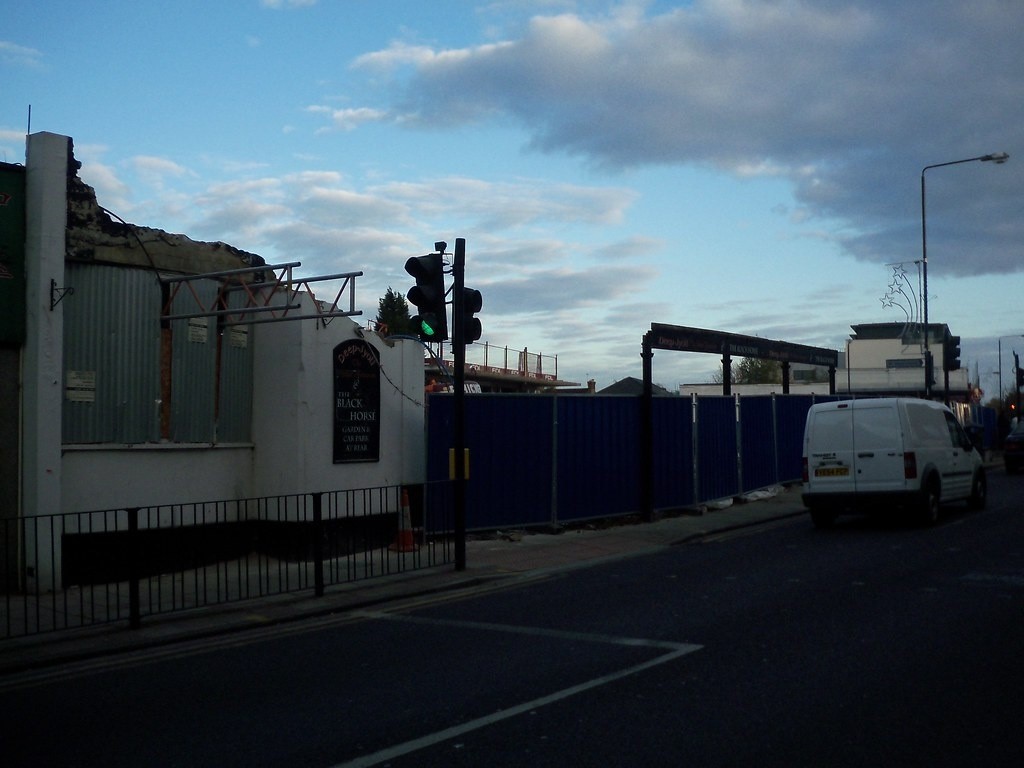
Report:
[388,489,423,553]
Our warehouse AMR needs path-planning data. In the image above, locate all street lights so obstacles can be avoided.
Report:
[998,334,1024,412]
[921,150,1011,399]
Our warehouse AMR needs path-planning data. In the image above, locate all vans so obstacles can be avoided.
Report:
[799,397,987,525]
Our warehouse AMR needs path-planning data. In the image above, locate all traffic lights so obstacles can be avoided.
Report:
[942,330,961,371]
[451,284,483,346]
[1017,367,1024,386]
[403,253,449,345]
[1008,402,1019,411]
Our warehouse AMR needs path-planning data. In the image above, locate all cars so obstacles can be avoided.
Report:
[1002,419,1024,470]
